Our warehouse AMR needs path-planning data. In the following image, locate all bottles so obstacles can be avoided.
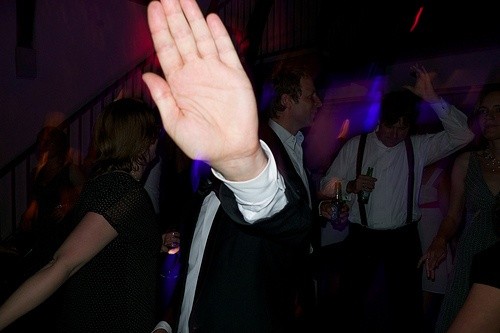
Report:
[331,183,344,227]
[357,167,374,204]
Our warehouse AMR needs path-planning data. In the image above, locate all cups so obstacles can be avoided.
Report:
[163,233,180,254]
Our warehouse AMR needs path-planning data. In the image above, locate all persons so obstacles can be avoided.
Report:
[416,84,500,333]
[319,62,473,333]
[0,97,162,333]
[259,65,349,260]
[141,1,320,333]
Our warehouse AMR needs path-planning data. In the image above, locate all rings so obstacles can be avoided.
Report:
[425,258,429,260]
